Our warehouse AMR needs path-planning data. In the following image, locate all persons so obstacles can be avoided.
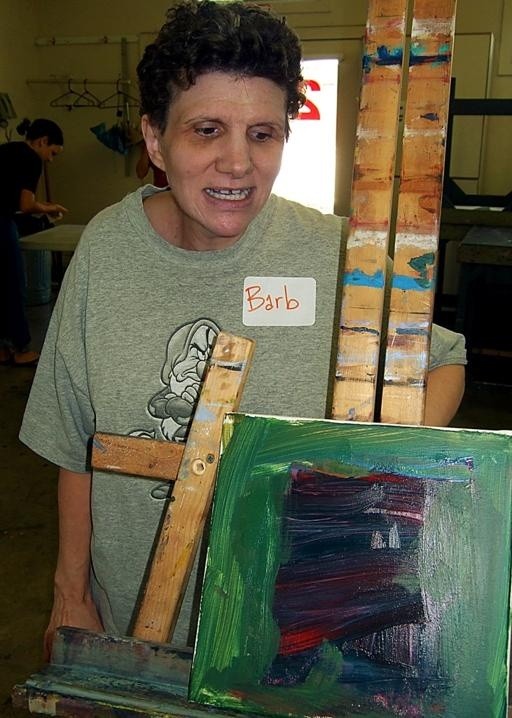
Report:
[18,0,473,663]
[0,115,71,367]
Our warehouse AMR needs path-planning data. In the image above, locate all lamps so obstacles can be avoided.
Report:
[0,92,18,141]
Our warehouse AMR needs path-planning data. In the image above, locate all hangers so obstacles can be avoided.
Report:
[96,78,142,109]
[49,78,95,107]
[72,78,105,107]
